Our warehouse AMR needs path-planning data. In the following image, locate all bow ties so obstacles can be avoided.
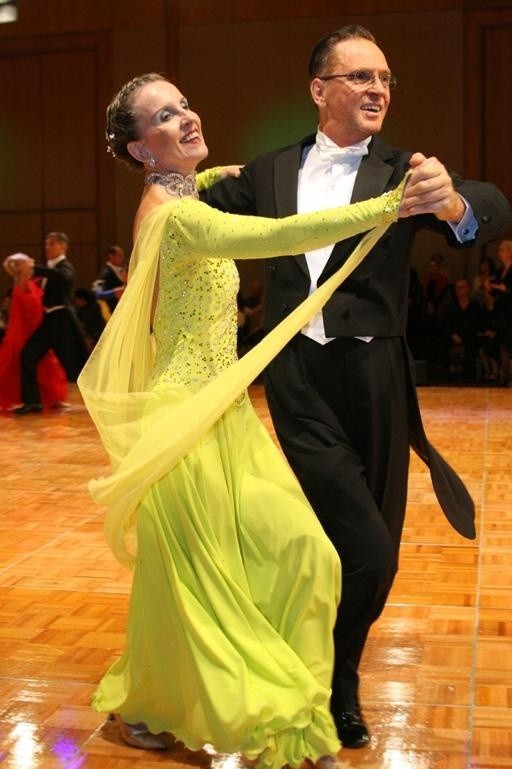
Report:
[319,144,369,161]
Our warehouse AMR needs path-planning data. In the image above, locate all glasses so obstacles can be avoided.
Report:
[316,70,399,92]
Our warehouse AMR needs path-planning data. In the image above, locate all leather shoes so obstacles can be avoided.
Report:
[14,403,43,414]
[326,682,372,750]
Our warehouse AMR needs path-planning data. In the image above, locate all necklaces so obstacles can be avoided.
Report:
[144,164,202,199]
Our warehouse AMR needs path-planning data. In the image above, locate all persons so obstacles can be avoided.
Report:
[74,70,454,767]
[141,25,512,749]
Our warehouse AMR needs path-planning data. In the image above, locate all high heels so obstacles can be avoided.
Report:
[107,712,173,752]
